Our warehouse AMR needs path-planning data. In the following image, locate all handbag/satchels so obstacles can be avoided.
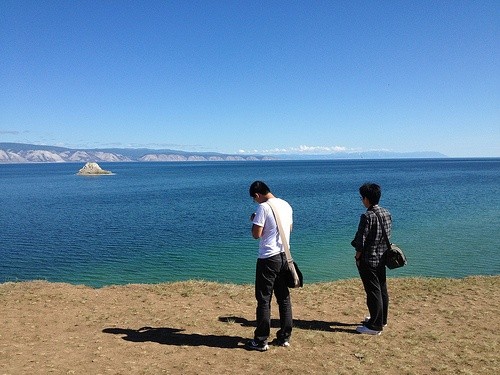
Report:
[285,260,303,288]
[385,245,407,270]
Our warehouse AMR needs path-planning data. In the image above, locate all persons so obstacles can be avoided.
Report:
[351,182,396,336]
[243,180,297,353]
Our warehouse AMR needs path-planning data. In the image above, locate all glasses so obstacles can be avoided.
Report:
[360,196,365,201]
[253,195,257,203]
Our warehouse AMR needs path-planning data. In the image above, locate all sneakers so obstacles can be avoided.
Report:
[245,337,270,351]
[356,325,381,336]
[273,338,289,347]
[365,316,388,328]
